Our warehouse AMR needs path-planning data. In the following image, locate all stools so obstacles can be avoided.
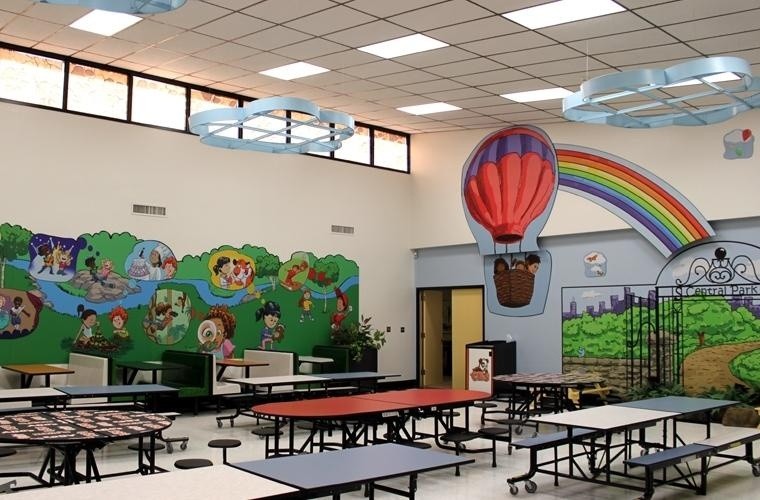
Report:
[174,459,211,469]
[208,438,241,463]
[380,417,399,438]
[129,443,164,464]
[2,448,16,458]
[252,428,284,458]
[438,410,462,427]
[480,426,506,469]
[300,423,325,447]
[442,431,479,475]
[474,403,496,424]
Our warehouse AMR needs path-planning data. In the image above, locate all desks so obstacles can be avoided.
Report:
[2,387,63,405]
[57,385,188,450]
[0,467,299,498]
[117,360,180,387]
[229,441,474,499]
[216,359,269,389]
[305,370,401,393]
[252,386,492,458]
[614,394,740,451]
[3,409,171,482]
[529,404,681,481]
[299,356,334,369]
[492,373,606,434]
[3,364,75,387]
[215,374,328,427]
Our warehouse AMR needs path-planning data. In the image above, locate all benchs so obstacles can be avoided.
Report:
[617,443,711,497]
[162,350,242,411]
[695,433,759,476]
[242,349,300,391]
[54,352,144,405]
[505,429,603,494]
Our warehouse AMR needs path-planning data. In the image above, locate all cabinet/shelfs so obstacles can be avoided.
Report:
[466,345,517,400]
